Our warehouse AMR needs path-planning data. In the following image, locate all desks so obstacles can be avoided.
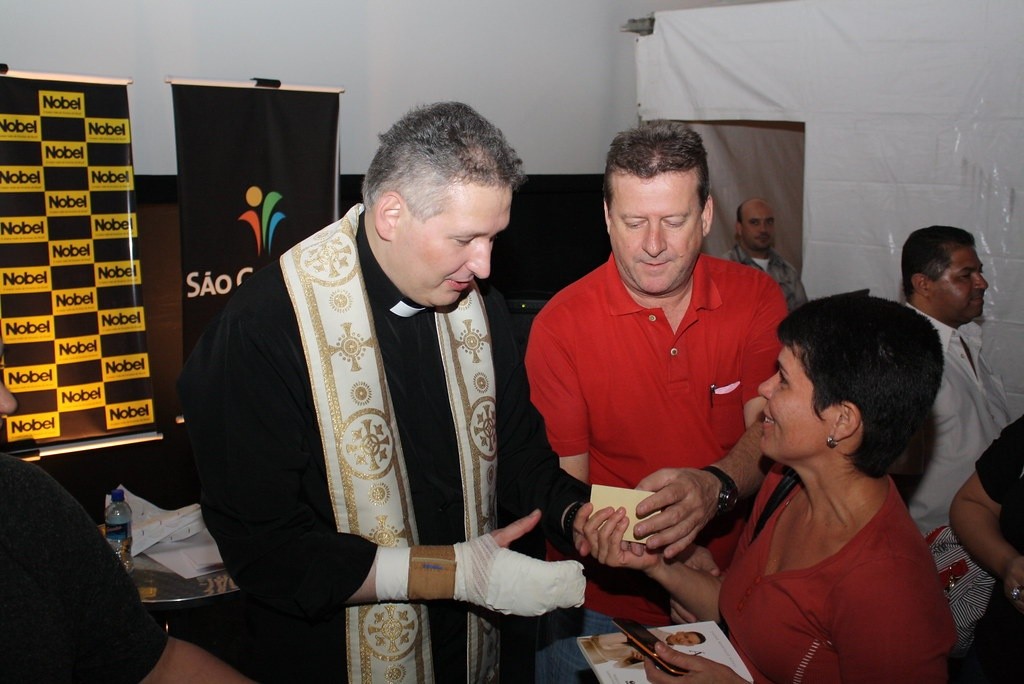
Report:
[129,554,241,637]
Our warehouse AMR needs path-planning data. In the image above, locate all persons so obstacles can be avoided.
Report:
[178,101,646,684]
[578,628,707,669]
[947,412,1024,684]
[899,226,1013,534]
[524,115,788,684]
[719,200,808,313]
[0,318,262,684]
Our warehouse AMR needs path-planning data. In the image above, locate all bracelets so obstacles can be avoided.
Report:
[560,501,586,535]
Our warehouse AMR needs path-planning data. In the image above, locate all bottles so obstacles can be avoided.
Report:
[105,489,132,556]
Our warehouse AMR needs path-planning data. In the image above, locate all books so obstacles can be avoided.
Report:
[576,620,753,684]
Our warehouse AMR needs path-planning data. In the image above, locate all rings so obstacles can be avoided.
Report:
[1011,585,1023,600]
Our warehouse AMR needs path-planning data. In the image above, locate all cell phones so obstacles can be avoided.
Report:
[612,618,689,677]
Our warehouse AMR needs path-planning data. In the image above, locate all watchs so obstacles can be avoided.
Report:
[700,464,740,512]
